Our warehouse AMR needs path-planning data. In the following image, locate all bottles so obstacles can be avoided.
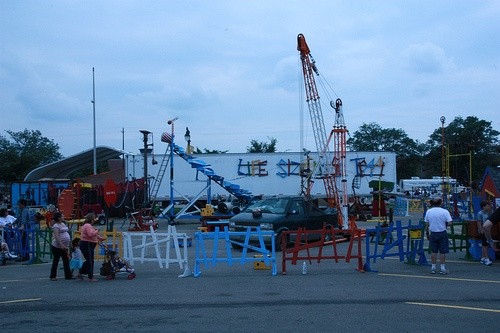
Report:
[5,221,8,228]
[302,262,307,274]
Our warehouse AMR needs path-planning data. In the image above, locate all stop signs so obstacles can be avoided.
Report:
[103,179,117,208]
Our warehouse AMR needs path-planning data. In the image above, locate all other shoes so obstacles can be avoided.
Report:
[51,278,56,281]
[65,277,76,280]
[76,275,84,280]
[89,278,98,282]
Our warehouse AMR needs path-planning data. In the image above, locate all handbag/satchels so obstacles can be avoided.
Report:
[100,261,112,276]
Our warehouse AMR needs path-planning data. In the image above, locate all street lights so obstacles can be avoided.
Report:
[184,126,193,153]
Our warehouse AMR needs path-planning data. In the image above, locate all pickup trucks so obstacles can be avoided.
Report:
[229,195,338,251]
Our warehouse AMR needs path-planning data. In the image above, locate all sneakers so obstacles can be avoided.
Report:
[480,258,485,263]
[431,268,437,274]
[485,258,492,265]
[439,268,449,275]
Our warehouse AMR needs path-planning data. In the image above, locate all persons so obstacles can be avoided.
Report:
[0,235,19,259]
[405,188,427,196]
[424,198,453,274]
[113,254,132,272]
[77,213,106,282]
[483,206,500,251]
[50,213,76,281]
[9,199,32,261]
[49,183,56,203]
[478,201,493,266]
[25,188,34,200]
[69,238,87,278]
[0,209,17,252]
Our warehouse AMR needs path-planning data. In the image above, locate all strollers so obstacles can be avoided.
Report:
[98,238,136,280]
